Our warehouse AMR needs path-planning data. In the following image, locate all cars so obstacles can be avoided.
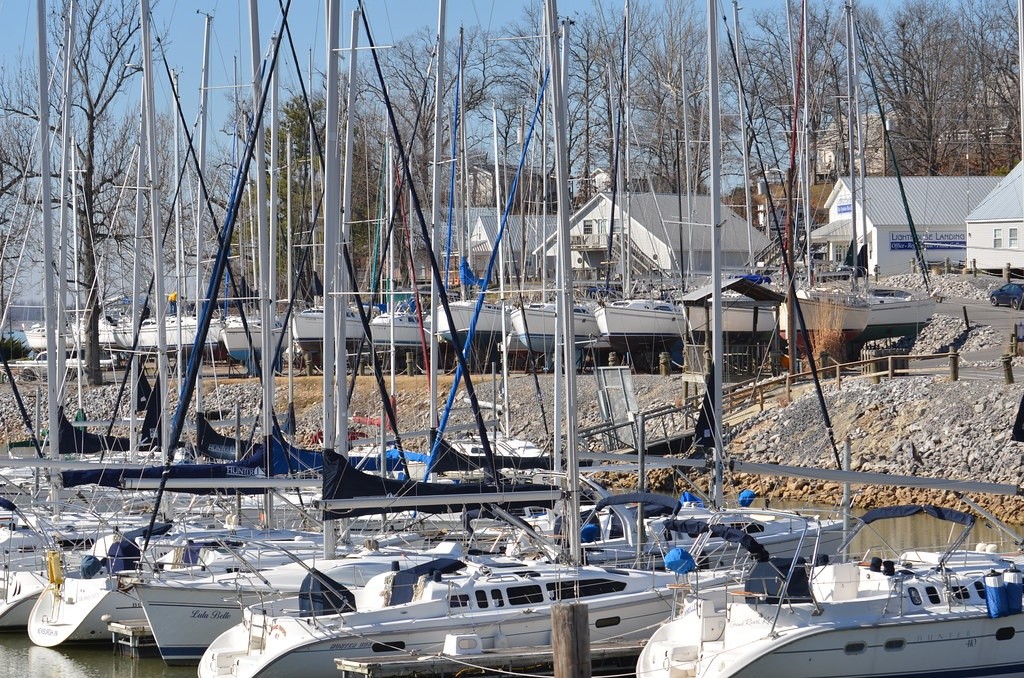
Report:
[989,283,1024,310]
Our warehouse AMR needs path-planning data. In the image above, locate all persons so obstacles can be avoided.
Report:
[169,290,180,315]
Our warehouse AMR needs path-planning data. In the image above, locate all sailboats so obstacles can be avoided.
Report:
[0,2,1024,678]
